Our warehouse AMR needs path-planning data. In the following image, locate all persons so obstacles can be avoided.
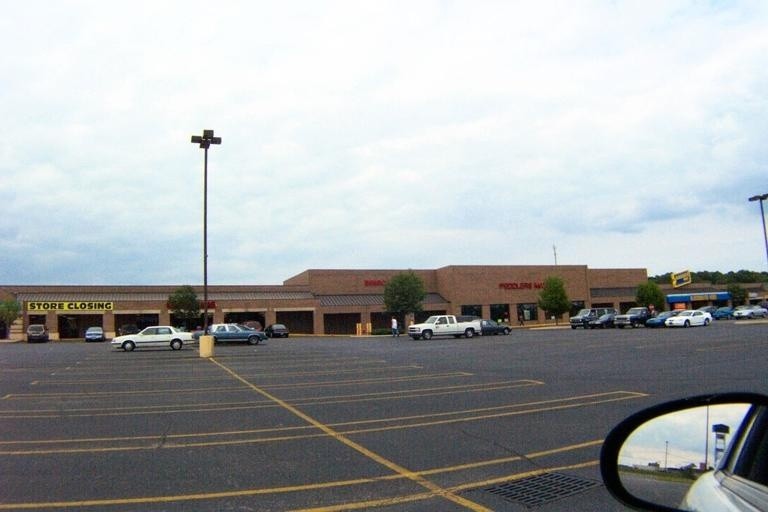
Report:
[391,316,399,337]
[520,316,524,326]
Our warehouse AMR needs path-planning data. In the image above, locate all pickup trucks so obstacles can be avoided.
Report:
[407,314,482,340]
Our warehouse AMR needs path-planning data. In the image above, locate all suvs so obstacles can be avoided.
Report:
[27,324,49,342]
[569,308,617,329]
[613,306,652,328]
[241,320,262,332]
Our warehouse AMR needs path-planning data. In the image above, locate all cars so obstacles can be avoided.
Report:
[480,319,512,335]
[697,306,718,318]
[733,304,767,320]
[712,306,735,320]
[194,320,263,346]
[456,316,481,325]
[265,323,289,338]
[646,310,682,328]
[118,323,141,336]
[110,325,195,351]
[678,402,768,511]
[665,309,712,328]
[85,326,106,342]
[588,313,616,329]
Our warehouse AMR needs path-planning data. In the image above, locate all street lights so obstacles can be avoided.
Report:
[748,193,768,262]
[191,127,221,332]
